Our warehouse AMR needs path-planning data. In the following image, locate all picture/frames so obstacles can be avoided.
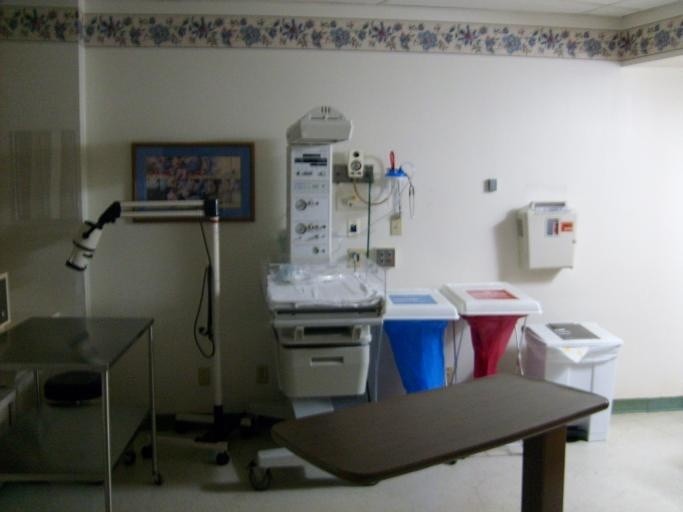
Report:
[131,141,256,222]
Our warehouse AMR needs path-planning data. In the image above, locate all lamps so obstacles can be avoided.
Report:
[66,196,251,466]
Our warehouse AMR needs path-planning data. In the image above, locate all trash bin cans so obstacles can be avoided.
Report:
[522,319,623,440]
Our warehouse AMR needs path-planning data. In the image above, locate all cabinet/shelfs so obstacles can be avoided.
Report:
[0,316,162,512]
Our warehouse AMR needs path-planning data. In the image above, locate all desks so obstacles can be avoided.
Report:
[270,370,609,512]
